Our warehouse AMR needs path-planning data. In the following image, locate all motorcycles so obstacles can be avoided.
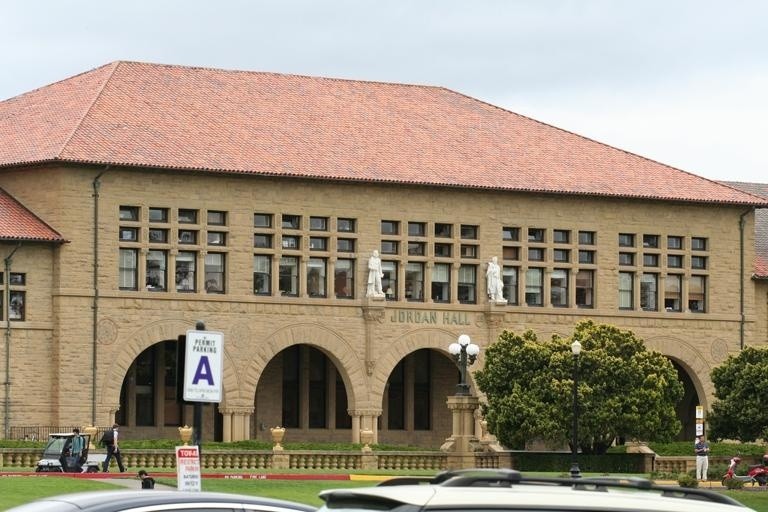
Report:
[721,450,768,489]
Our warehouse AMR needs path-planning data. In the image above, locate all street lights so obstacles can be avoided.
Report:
[446,334,481,397]
[569,341,586,473]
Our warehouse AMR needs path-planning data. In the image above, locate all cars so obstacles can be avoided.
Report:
[2,490,322,512]
[324,469,755,511]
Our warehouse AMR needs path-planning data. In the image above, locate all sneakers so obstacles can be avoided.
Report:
[121,468,128,473]
[698,480,706,482]
[102,470,109,473]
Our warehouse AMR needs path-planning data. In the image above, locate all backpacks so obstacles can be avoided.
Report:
[72,436,85,450]
[102,429,118,445]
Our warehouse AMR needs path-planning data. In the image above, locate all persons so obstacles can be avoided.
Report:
[694,435,710,481]
[102,423,127,473]
[139,470,155,489]
[367,250,385,296]
[71,429,87,474]
[485,256,506,302]
